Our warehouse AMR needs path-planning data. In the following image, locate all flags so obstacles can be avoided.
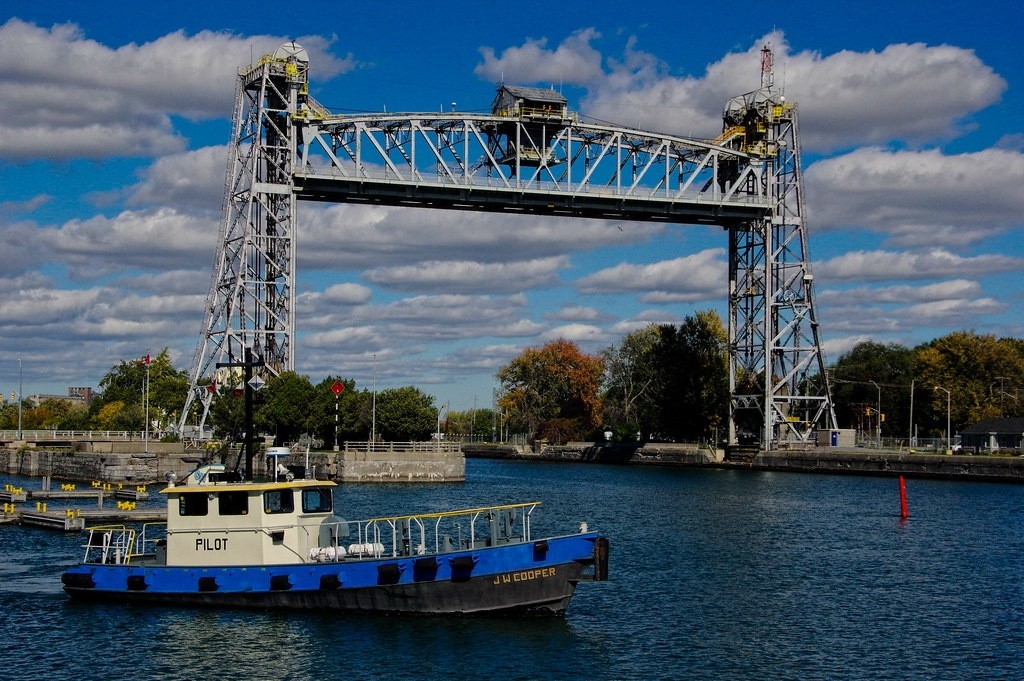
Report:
[140,354,150,364]
[207,367,216,393]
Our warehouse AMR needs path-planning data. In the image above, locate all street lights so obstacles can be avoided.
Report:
[935,387,951,449]
[11,358,23,440]
[869,380,881,446]
[437,404,446,449]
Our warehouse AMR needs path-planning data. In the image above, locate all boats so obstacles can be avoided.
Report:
[59,344,612,622]
